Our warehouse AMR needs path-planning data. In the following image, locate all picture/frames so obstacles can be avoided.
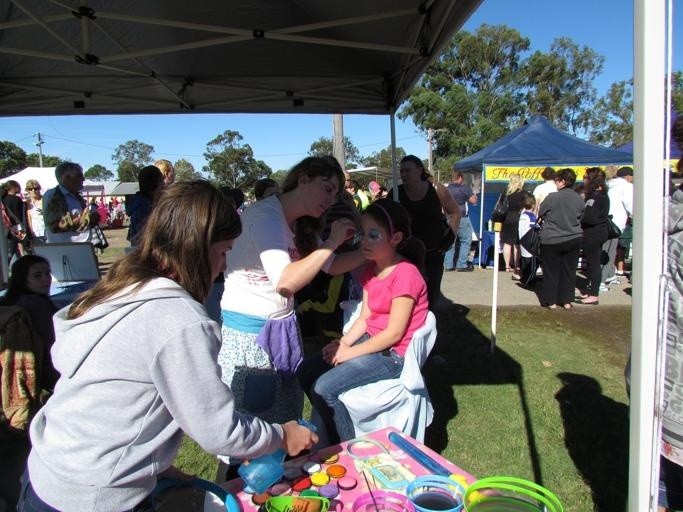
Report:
[32,242,101,283]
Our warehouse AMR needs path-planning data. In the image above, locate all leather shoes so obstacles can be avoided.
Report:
[576,299,598,304]
[575,296,585,299]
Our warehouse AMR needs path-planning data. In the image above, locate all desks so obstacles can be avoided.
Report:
[203,428,506,512]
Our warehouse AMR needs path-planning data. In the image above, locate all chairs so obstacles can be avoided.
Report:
[340,302,436,442]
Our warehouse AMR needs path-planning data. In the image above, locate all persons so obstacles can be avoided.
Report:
[206,186,245,326]
[27,180,49,244]
[321,189,357,332]
[120,198,126,214]
[42,161,101,243]
[216,155,371,487]
[578,167,610,304]
[153,159,175,185]
[387,155,462,312]
[498,174,532,273]
[125,165,165,247]
[445,170,479,273]
[615,214,632,277]
[343,179,361,212]
[293,210,358,373]
[254,178,281,202]
[624,118,683,512]
[518,193,541,290]
[532,168,559,219]
[17,179,320,512]
[351,180,369,210]
[89,195,99,212]
[538,168,585,310]
[0,184,23,291]
[0,254,61,512]
[111,196,119,208]
[5,180,31,264]
[368,176,389,202]
[298,198,429,446]
[598,166,634,293]
[109,198,115,214]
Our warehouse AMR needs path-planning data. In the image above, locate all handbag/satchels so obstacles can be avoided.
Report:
[607,215,620,240]
[520,216,540,257]
[491,192,507,223]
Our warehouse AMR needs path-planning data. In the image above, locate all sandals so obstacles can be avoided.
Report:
[505,266,514,272]
